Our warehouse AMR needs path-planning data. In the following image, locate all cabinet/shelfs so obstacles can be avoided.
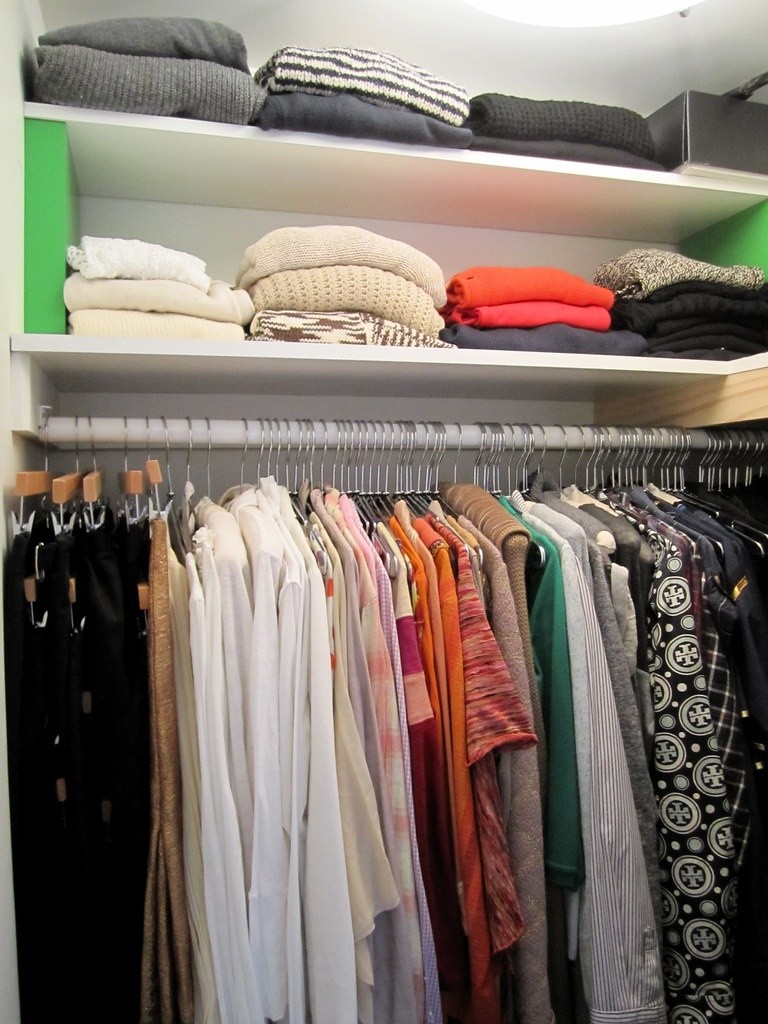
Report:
[12,102,768,396]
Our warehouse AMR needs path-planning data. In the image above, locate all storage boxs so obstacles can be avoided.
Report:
[644,88,768,184]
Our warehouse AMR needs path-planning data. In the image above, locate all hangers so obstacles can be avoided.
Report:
[13,408,768,633]
